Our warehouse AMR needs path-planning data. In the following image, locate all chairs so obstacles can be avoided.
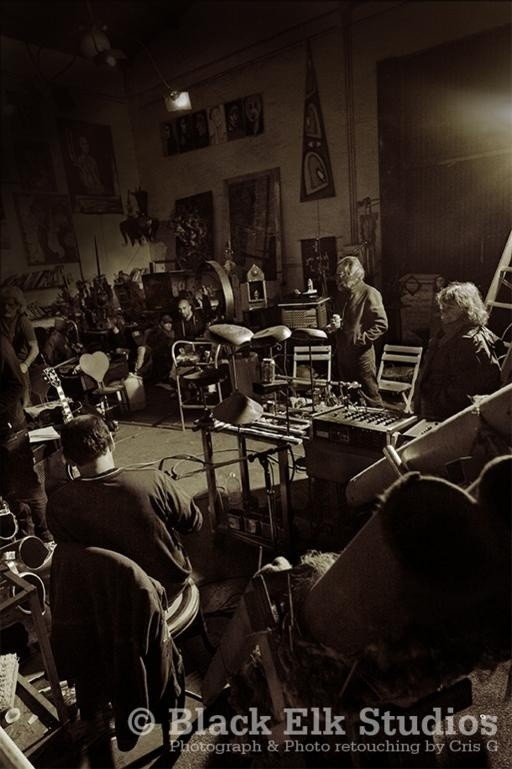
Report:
[377,343,423,415]
[171,340,222,432]
[292,343,332,398]
[79,350,133,423]
[50,545,201,768]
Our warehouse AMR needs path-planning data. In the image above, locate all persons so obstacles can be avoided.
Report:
[415,281,505,423]
[76,136,105,196]
[0,287,212,543]
[326,256,389,408]
[160,93,261,156]
[45,414,203,613]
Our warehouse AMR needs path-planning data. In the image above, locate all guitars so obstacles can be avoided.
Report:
[41,367,83,479]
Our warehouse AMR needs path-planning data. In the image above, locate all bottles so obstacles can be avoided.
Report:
[302,277,318,299]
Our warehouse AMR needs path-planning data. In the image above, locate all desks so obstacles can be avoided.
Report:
[0,561,63,706]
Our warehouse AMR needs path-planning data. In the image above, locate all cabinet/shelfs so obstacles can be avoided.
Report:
[278,297,331,330]
